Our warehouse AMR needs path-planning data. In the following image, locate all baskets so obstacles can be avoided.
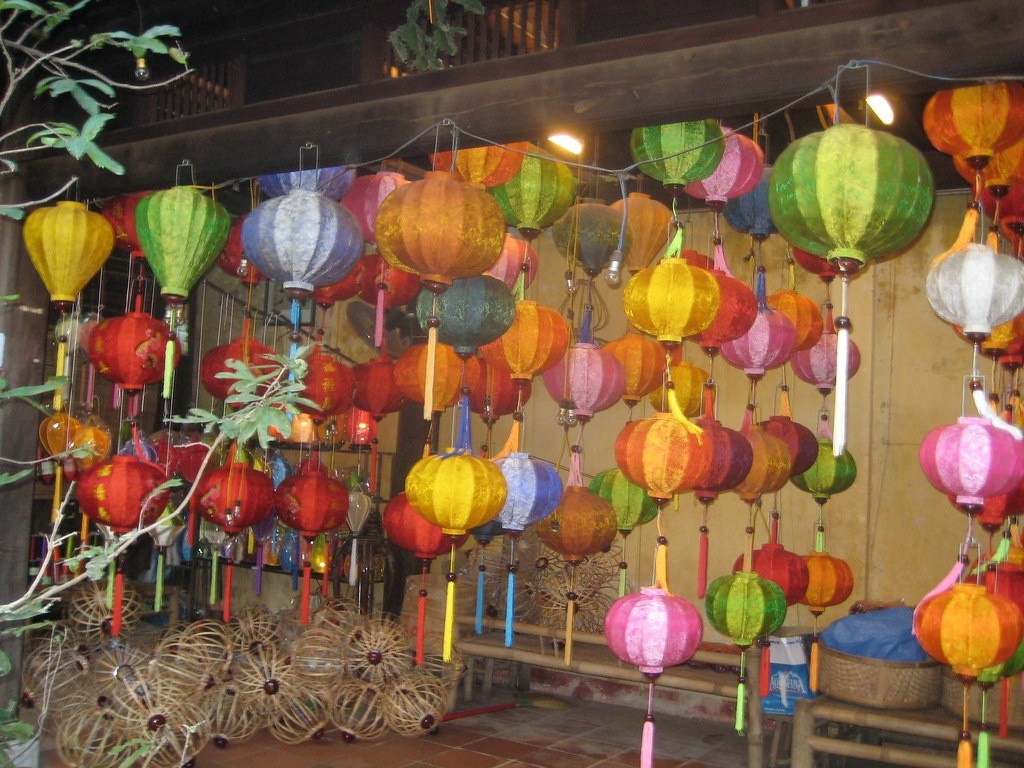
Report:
[816,639,1023,730]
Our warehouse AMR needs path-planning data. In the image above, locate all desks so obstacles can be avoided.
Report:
[791,696,1024,768]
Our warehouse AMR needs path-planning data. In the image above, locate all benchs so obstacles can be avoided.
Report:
[446,614,763,768]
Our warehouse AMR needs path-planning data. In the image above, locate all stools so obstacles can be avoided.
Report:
[767,714,830,768]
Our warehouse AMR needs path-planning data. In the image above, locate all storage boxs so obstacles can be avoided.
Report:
[400,573,491,657]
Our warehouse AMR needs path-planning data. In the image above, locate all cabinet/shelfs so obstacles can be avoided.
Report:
[187,445,395,622]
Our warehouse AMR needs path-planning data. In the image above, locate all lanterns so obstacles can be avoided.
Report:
[22,82,1024,768]
